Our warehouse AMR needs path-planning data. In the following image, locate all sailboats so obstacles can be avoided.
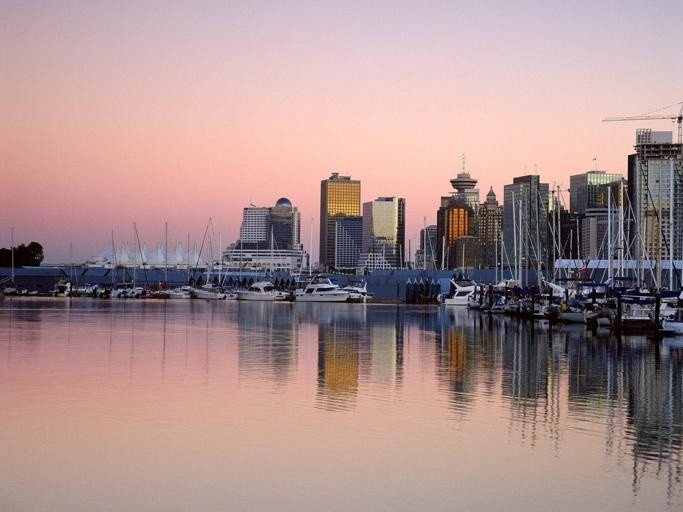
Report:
[442,184,682,335]
[0,216,376,304]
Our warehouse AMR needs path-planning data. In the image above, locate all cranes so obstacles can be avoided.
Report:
[602,101,682,145]
